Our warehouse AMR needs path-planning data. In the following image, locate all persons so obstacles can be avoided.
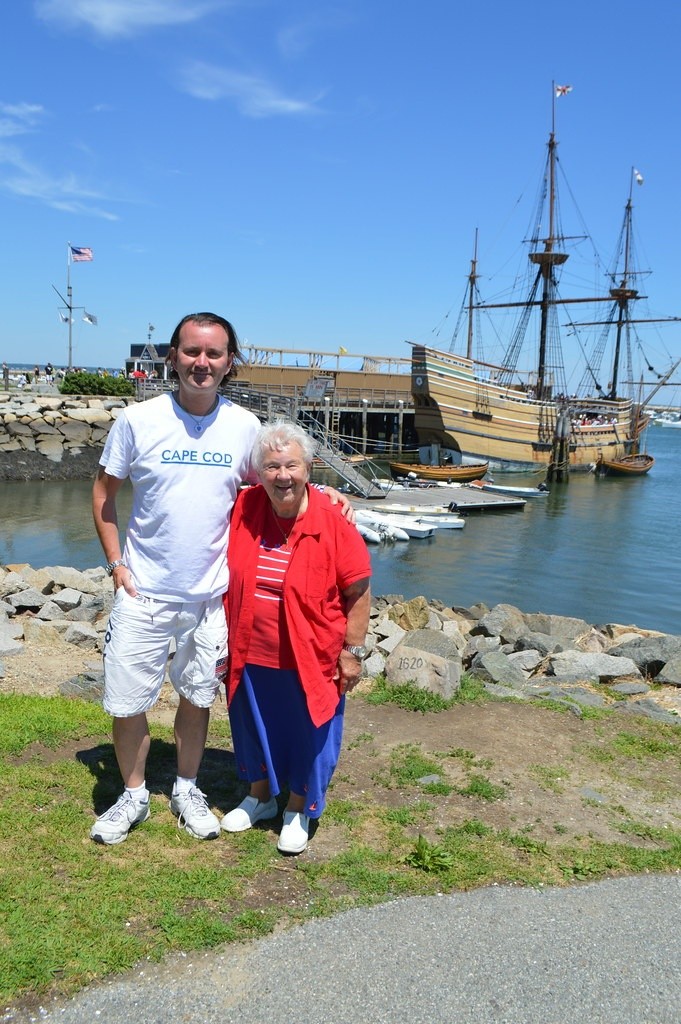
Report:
[169,367,176,391]
[572,414,617,426]
[57,366,86,378]
[17,373,30,387]
[221,420,373,852]
[34,365,39,384]
[44,363,55,385]
[88,312,355,845]
[97,367,158,389]
[2,361,6,378]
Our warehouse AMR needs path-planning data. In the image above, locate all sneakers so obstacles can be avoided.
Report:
[167,782,221,840]
[220,795,278,831]
[89,790,152,844]
[277,806,309,853]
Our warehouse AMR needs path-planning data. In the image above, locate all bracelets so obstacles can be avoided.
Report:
[310,483,326,493]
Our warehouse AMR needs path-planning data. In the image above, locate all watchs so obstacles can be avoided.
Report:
[344,645,367,658]
[106,560,124,577]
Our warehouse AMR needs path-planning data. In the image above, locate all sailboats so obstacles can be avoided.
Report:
[602,373,655,476]
[411,79,680,472]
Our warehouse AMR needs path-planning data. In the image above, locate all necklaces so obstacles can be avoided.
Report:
[272,509,298,546]
[176,390,217,433]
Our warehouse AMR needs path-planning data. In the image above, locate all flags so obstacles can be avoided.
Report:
[71,247,93,261]
[634,170,643,184]
[556,85,572,97]
[83,312,95,324]
[60,313,74,325]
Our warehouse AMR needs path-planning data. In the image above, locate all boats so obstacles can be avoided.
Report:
[309,454,373,469]
[372,472,551,498]
[389,461,489,482]
[347,502,465,543]
